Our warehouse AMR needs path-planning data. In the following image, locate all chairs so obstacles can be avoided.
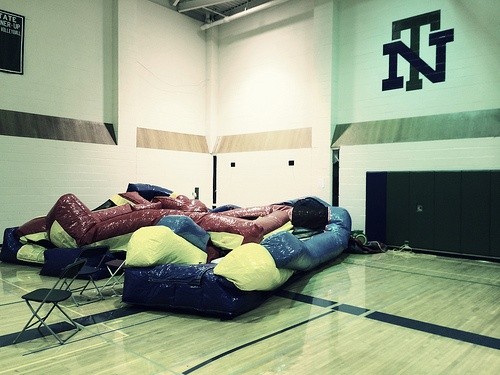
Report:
[12,260,88,346]
[96,253,126,296]
[60,245,109,307]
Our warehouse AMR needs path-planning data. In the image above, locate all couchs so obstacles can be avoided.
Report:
[1,182,352,320]
